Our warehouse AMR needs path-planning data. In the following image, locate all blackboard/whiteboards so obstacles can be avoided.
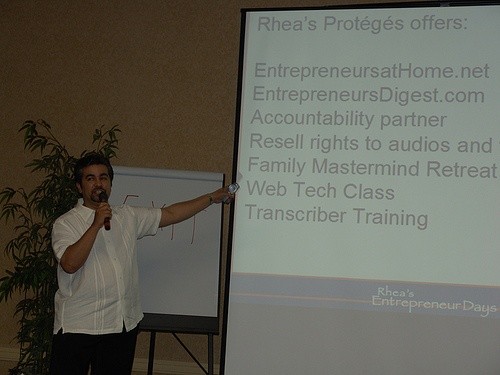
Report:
[105,165,226,335]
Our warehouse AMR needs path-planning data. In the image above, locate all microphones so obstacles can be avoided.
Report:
[99,192,110,230]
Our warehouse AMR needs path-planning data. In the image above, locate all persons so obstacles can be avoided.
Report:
[51,151,231,375]
